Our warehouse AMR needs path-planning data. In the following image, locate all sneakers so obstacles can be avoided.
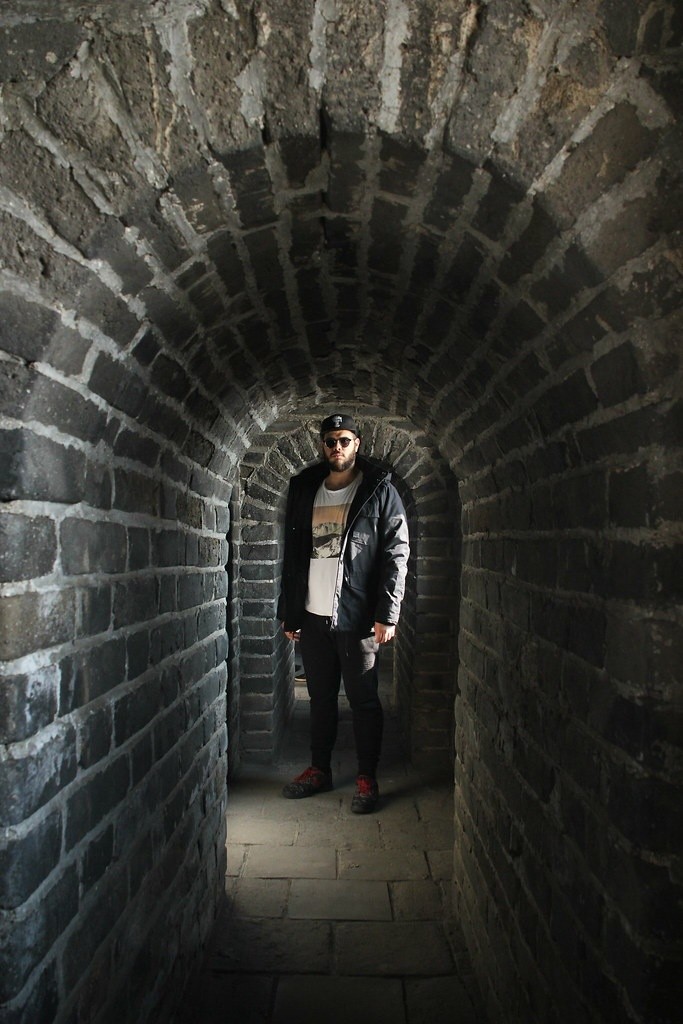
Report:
[351,775,379,812]
[283,767,334,798]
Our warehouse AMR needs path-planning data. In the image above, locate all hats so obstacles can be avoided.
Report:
[320,414,356,439]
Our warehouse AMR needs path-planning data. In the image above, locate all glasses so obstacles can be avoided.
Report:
[324,437,351,447]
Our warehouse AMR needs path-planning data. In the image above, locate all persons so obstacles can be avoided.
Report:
[277,413,410,813]
